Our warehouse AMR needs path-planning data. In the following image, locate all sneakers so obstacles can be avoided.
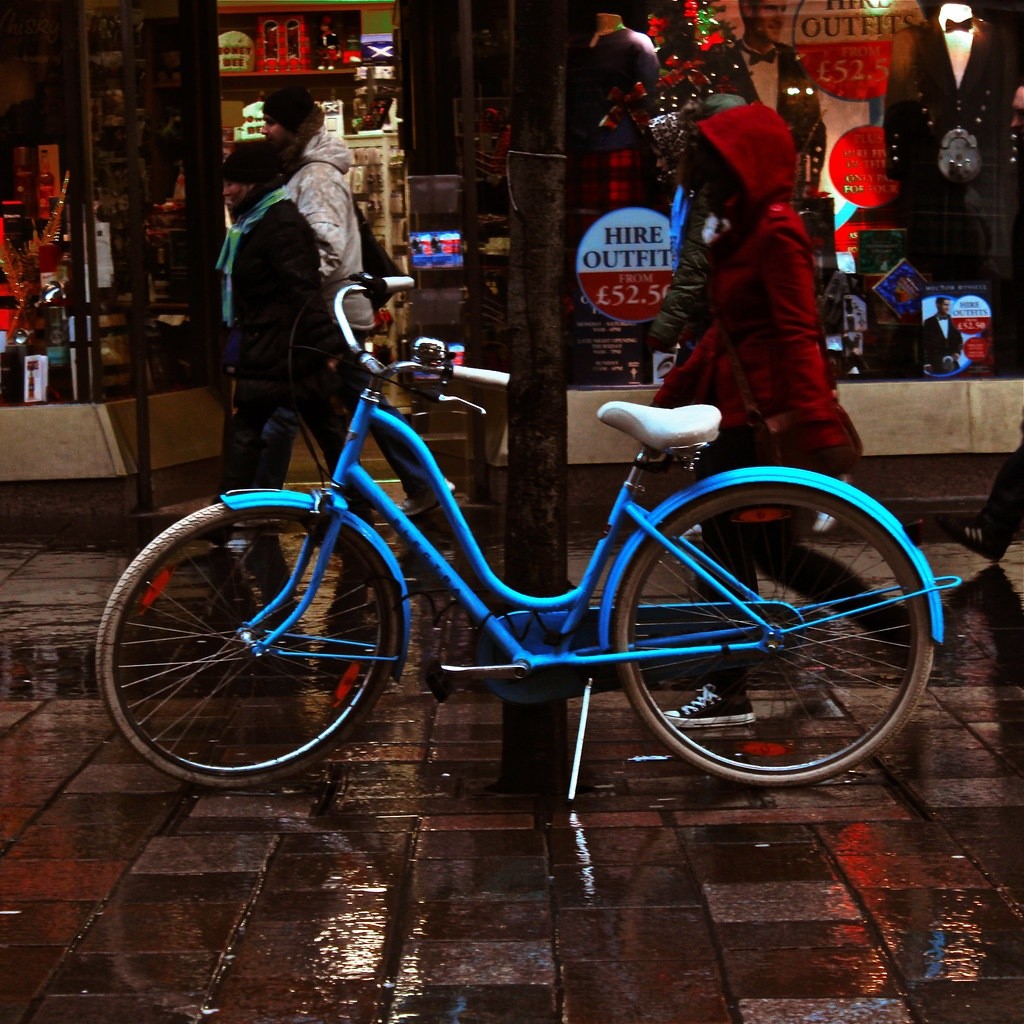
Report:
[213,525,275,553]
[396,477,455,519]
[663,687,756,728]
[948,565,1004,609]
[933,512,1014,562]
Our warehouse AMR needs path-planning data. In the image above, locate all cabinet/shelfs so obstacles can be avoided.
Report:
[216,3,402,153]
[340,132,414,418]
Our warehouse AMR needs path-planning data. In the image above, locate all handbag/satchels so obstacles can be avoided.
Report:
[760,402,865,486]
[354,202,407,311]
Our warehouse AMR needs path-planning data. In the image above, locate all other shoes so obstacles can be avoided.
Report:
[350,503,375,529]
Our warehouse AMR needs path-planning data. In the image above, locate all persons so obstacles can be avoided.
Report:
[924,298,963,374]
[573,13,658,211]
[657,102,912,728]
[733,0,827,183]
[888,2,1001,270]
[937,82,1024,562]
[200,83,454,637]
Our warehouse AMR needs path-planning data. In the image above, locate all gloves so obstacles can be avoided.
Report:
[648,337,666,352]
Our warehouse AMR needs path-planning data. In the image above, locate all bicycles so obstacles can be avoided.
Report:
[95,272,962,789]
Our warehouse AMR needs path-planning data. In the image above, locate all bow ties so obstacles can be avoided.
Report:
[945,17,974,32]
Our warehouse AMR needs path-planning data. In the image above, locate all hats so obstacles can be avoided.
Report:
[214,142,281,184]
[263,83,313,134]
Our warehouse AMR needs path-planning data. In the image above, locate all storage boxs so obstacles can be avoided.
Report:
[22,354,51,403]
[858,228,909,275]
[864,274,935,327]
